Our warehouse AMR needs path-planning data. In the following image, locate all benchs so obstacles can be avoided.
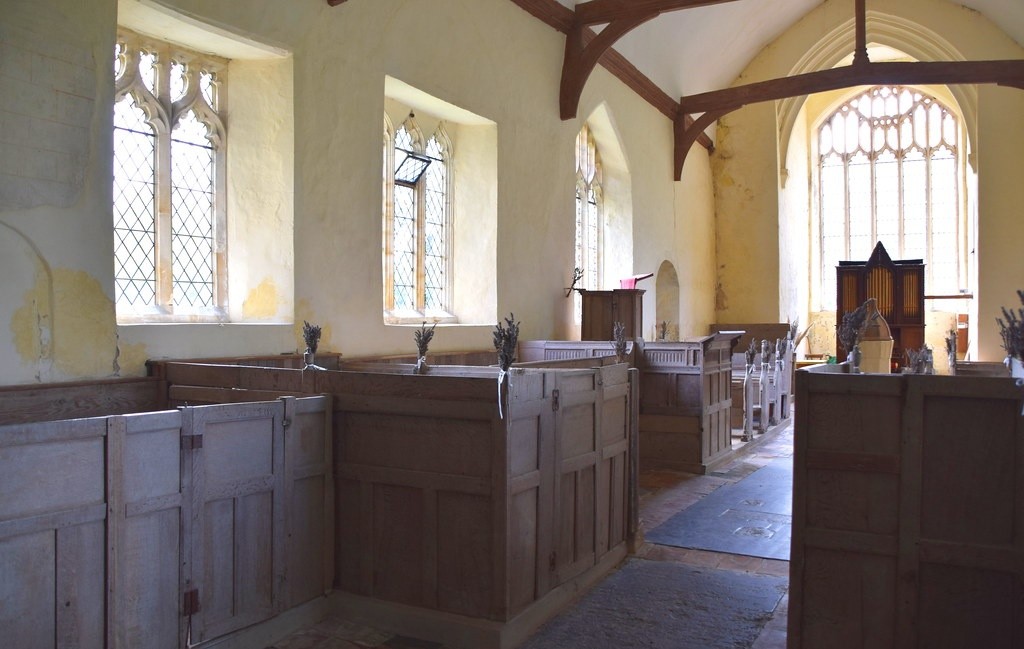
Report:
[731,351,785,442]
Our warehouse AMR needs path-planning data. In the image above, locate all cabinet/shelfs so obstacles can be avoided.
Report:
[578,287,646,340]
